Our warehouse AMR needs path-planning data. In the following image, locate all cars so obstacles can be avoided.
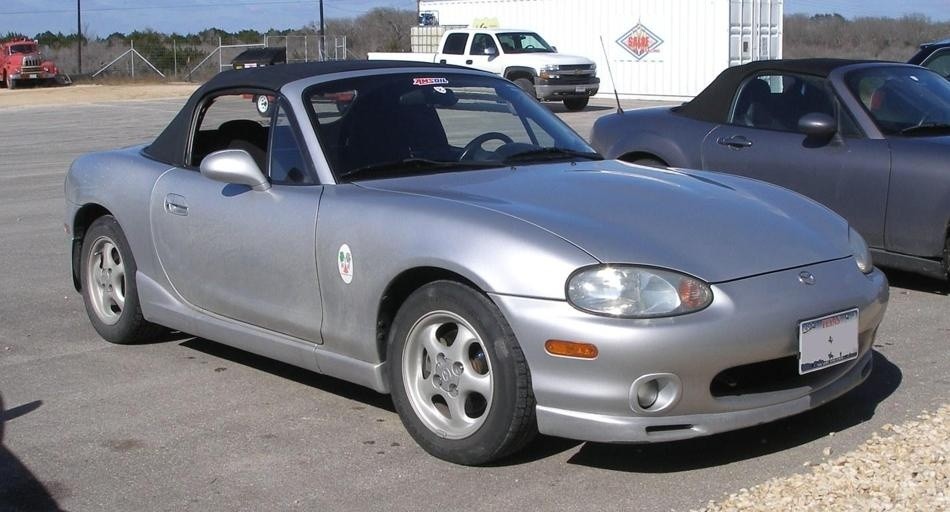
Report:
[591,58,950,288]
[63,62,890,465]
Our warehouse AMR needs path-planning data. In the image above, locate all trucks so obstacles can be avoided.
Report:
[0,39,57,89]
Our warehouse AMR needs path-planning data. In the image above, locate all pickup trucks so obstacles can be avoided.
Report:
[365,27,601,111]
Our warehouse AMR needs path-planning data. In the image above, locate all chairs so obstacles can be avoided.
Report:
[216,102,449,180]
[735,80,922,129]
